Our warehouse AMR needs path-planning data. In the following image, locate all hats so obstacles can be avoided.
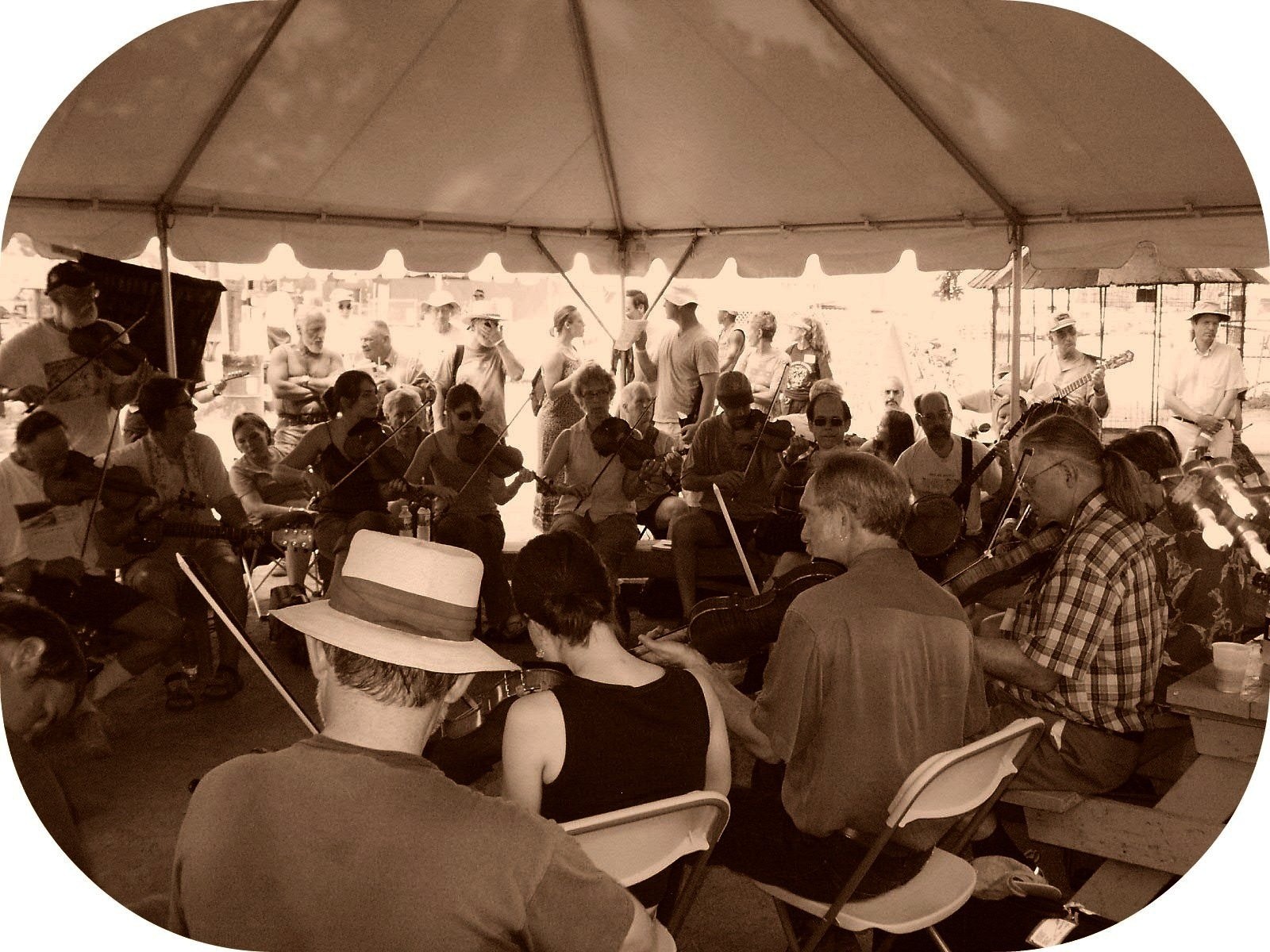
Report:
[420,290,462,317]
[462,300,508,325]
[44,261,94,295]
[1186,300,1231,322]
[329,289,353,303]
[1044,311,1077,333]
[781,315,812,331]
[715,370,754,408]
[267,529,523,672]
[471,289,485,298]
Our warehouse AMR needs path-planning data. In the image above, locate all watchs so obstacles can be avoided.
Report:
[39,561,47,576]
[302,375,311,386]
[1004,463,1016,473]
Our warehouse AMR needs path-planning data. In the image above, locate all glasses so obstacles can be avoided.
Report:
[173,396,194,408]
[336,304,353,310]
[812,418,847,427]
[76,290,100,304]
[919,413,952,421]
[451,410,485,421]
[479,323,500,327]
[1018,462,1061,496]
[577,391,610,400]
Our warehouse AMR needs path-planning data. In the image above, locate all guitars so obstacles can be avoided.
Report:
[1022,350,1135,404]
[901,403,1041,561]
[93,505,315,553]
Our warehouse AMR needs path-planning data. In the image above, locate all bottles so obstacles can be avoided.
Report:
[417,502,430,541]
[398,505,413,537]
[1262,600,1270,665]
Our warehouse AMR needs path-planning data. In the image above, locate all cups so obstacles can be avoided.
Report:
[1212,642,1252,694]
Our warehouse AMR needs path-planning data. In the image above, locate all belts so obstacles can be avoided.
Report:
[1172,416,1199,426]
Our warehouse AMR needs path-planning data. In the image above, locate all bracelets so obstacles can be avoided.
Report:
[637,474,645,482]
[398,382,404,390]
[286,506,297,520]
[1200,433,1214,442]
[496,339,504,347]
[636,347,647,354]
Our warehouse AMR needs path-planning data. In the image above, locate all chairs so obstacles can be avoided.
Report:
[241,545,325,621]
[749,716,1045,952]
[559,791,732,941]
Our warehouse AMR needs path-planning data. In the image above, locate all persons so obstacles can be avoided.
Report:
[166,530,688,952]
[0,261,1251,919]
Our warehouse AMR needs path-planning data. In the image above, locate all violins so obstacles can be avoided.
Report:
[189,746,280,795]
[621,556,847,665]
[346,420,432,510]
[43,449,206,512]
[456,423,557,497]
[732,409,818,452]
[590,416,682,493]
[937,521,1066,608]
[421,662,572,785]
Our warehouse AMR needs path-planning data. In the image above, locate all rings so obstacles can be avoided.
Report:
[1004,450,1007,455]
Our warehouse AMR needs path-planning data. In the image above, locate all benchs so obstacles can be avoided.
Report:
[501,538,774,578]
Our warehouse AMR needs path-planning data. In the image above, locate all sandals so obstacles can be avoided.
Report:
[202,666,245,701]
[163,672,196,711]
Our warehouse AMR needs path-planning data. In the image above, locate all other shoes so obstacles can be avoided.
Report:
[71,710,115,759]
